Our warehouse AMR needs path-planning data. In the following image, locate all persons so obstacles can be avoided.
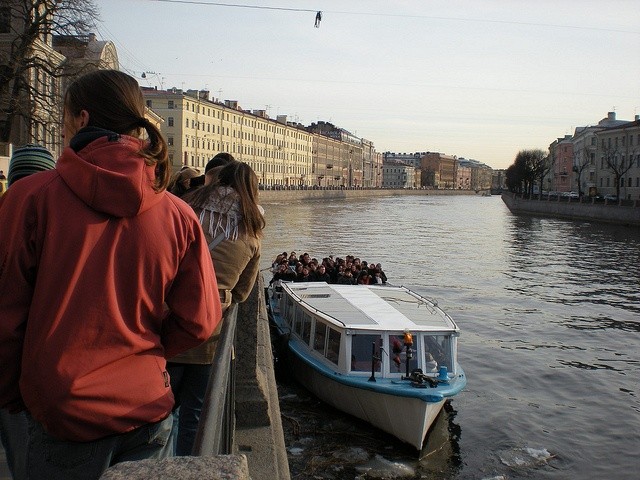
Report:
[164,161,262,459]
[0,146,55,478]
[0,68,223,479]
[269,252,387,289]
[167,169,200,197]
[180,153,236,202]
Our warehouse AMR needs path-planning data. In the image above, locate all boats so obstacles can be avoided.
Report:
[266,281,467,451]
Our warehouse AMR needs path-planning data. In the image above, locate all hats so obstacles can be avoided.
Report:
[7,141,55,187]
